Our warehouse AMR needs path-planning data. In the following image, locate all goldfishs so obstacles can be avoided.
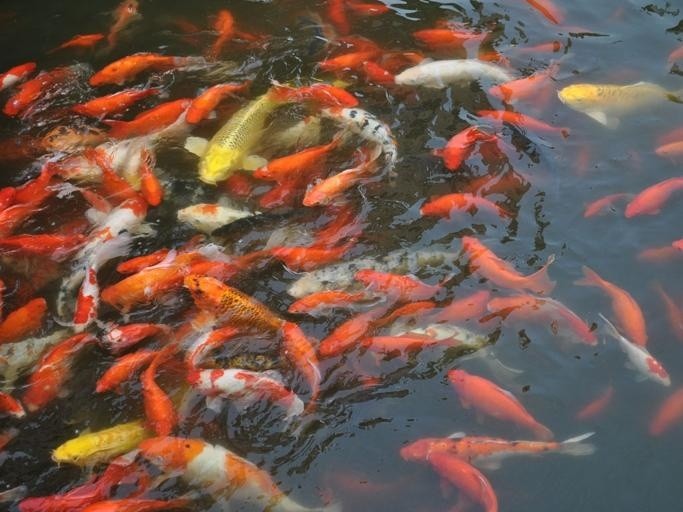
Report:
[1,1,683,508]
